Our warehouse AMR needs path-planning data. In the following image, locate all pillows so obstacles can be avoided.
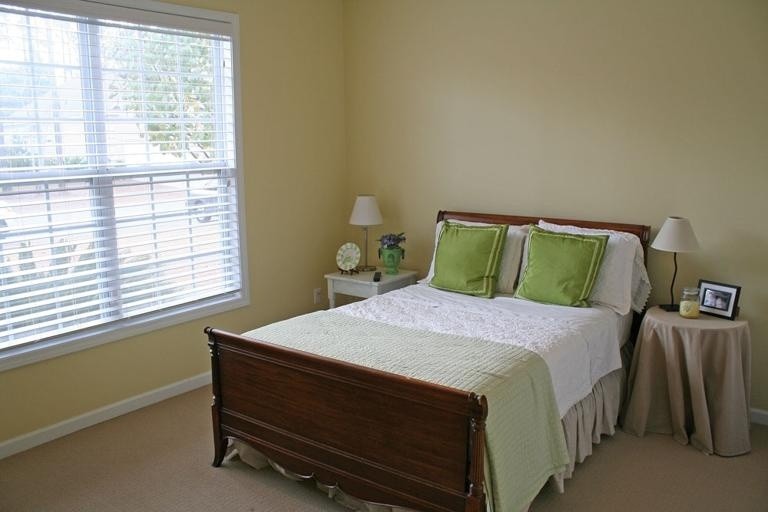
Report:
[417,218,645,316]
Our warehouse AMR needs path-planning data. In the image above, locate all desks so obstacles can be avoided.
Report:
[646,305,747,446]
[324,268,418,308]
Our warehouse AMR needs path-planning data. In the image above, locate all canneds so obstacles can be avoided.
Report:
[679,288,700,319]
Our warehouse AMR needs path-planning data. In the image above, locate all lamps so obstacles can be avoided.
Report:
[650,217,700,312]
[349,196,384,270]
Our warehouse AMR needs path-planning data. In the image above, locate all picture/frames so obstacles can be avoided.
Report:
[698,279,741,321]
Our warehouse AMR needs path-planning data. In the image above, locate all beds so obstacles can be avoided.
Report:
[203,213,647,512]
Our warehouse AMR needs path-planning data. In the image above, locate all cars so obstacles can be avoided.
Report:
[184,178,235,223]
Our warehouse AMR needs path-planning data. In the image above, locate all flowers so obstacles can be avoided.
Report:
[381,233,405,248]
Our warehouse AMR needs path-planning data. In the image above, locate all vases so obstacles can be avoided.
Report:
[379,249,403,274]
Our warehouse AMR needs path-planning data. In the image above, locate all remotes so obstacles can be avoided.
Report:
[374,272,381,281]
